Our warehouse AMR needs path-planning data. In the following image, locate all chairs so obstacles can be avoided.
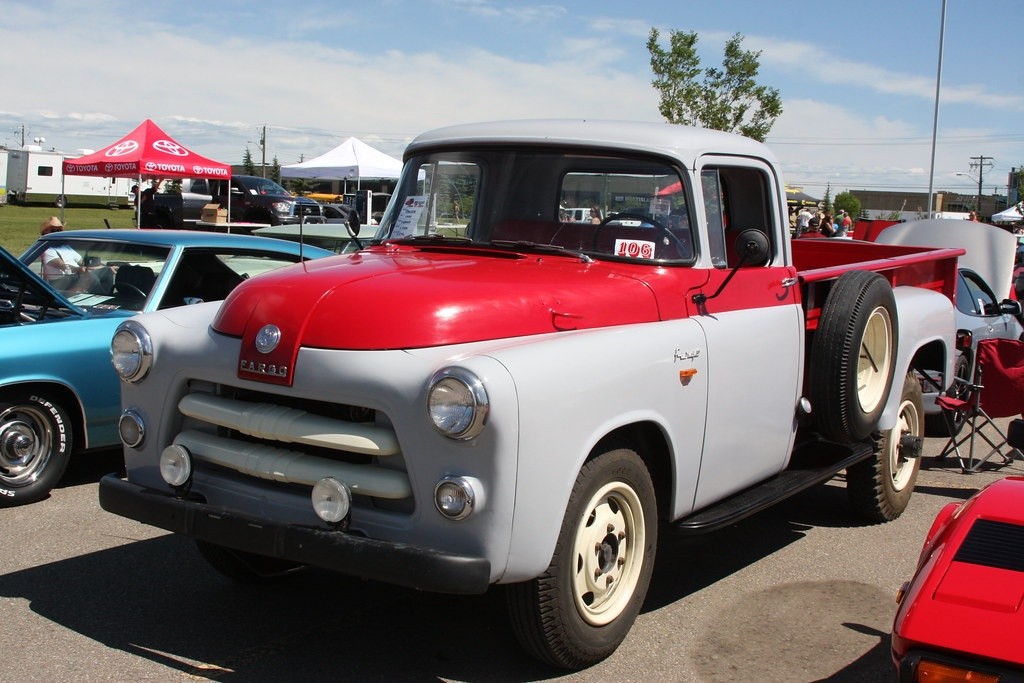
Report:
[155,274,205,313]
[932,338,1023,474]
[111,265,154,300]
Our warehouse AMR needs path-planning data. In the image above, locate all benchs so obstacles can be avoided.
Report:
[489,218,755,273]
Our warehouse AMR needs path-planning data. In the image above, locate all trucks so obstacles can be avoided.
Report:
[5,148,155,208]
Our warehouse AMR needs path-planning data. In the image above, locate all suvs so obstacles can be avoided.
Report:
[159,177,213,221]
[191,174,328,230]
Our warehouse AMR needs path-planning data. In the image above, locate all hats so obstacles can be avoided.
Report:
[809,218,819,226]
[131,185,138,192]
[40,217,63,235]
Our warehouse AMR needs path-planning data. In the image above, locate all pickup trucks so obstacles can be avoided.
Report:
[96,115,966,667]
[561,207,643,227]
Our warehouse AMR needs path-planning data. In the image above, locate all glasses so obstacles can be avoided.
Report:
[133,190,137,193]
[590,210,595,213]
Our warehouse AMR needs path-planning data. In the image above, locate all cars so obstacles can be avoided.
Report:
[873,218,1024,436]
[321,202,360,225]
[251,224,446,254]
[889,477,1023,683]
[0,227,339,498]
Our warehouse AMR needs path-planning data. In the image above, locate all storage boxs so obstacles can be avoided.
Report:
[203,203,227,223]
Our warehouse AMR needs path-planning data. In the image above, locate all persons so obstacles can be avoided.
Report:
[965,211,978,222]
[788,204,851,237]
[40,217,114,294]
[130,178,164,207]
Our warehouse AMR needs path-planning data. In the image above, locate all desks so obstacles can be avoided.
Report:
[196,221,271,237]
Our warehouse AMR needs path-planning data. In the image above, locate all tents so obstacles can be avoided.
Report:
[280,136,426,197]
[992,201,1024,224]
[60,119,230,233]
